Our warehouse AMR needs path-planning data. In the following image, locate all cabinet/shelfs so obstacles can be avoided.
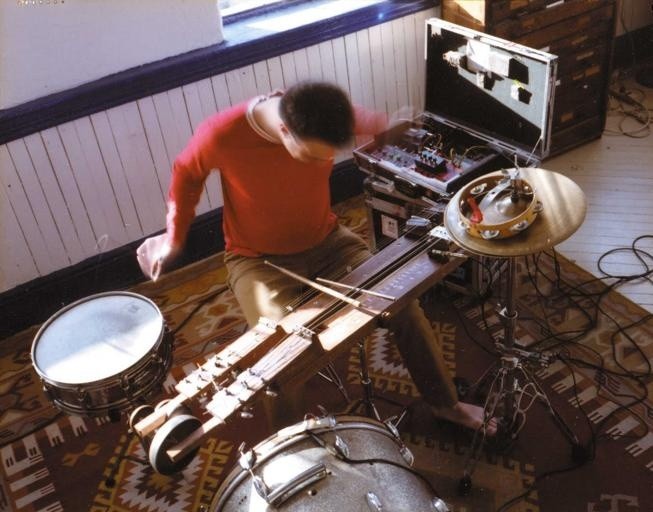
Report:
[441,0,621,158]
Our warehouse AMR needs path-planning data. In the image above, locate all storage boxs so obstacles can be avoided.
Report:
[359,176,508,297]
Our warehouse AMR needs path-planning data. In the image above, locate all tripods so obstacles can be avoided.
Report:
[347,346,406,425]
[453,259,584,495]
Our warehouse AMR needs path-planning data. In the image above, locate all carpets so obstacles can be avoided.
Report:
[0,189,653,512]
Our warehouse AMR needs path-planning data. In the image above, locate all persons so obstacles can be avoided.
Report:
[136,82,499,437]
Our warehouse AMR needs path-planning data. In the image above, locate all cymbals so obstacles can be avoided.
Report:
[444,167,589,256]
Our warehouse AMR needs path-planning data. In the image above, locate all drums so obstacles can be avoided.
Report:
[207,405,450,512]
[30,291,173,415]
